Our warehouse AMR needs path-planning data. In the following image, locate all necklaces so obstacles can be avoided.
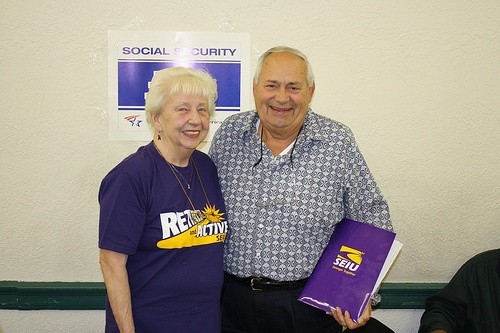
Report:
[152,138,219,223]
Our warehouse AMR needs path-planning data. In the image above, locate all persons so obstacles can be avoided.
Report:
[208,46,393,333]
[418,249,500,333]
[98,66,230,333]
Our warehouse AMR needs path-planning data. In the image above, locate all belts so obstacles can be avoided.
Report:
[224,273,309,291]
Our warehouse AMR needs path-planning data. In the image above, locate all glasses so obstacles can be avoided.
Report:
[252,166,298,206]
[191,211,221,223]
